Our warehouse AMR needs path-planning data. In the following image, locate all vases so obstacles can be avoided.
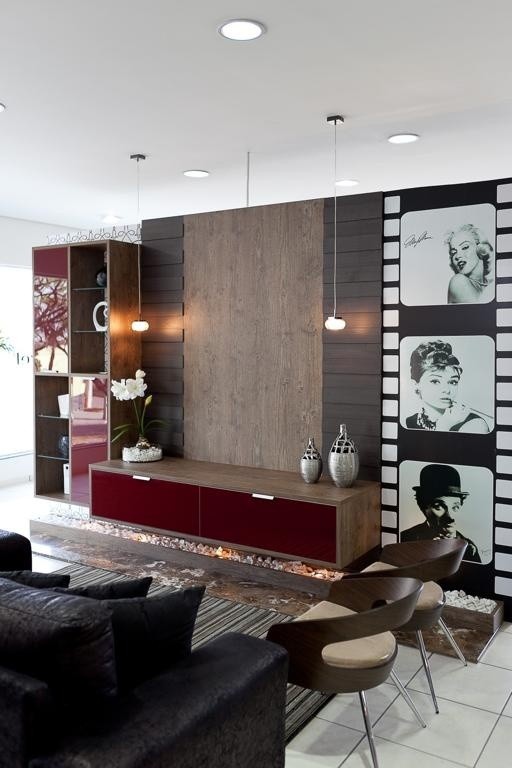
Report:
[121,443,163,463]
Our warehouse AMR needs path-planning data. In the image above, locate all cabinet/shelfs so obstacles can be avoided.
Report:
[89,455,382,570]
[32,240,144,514]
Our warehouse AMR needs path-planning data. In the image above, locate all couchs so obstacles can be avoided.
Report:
[0,528,288,768]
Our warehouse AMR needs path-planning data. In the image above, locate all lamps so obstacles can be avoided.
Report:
[325,116,346,331]
[131,154,149,332]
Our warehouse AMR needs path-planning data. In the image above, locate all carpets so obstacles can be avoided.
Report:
[46,562,339,747]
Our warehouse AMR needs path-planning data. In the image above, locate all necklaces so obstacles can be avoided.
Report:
[469,276,489,286]
[417,406,440,430]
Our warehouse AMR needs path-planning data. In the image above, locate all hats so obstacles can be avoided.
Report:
[413,464,472,497]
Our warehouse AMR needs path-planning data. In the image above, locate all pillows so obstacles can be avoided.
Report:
[0,577,121,734]
[1,569,71,590]
[43,576,153,601]
[103,584,206,710]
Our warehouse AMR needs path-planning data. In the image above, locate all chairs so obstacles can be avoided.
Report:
[265,576,426,768]
[322,538,469,714]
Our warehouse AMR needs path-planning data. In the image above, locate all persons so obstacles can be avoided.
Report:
[443,220,497,306]
[399,462,483,563]
[403,340,490,434]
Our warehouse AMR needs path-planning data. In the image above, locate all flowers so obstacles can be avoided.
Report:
[111,370,166,449]
